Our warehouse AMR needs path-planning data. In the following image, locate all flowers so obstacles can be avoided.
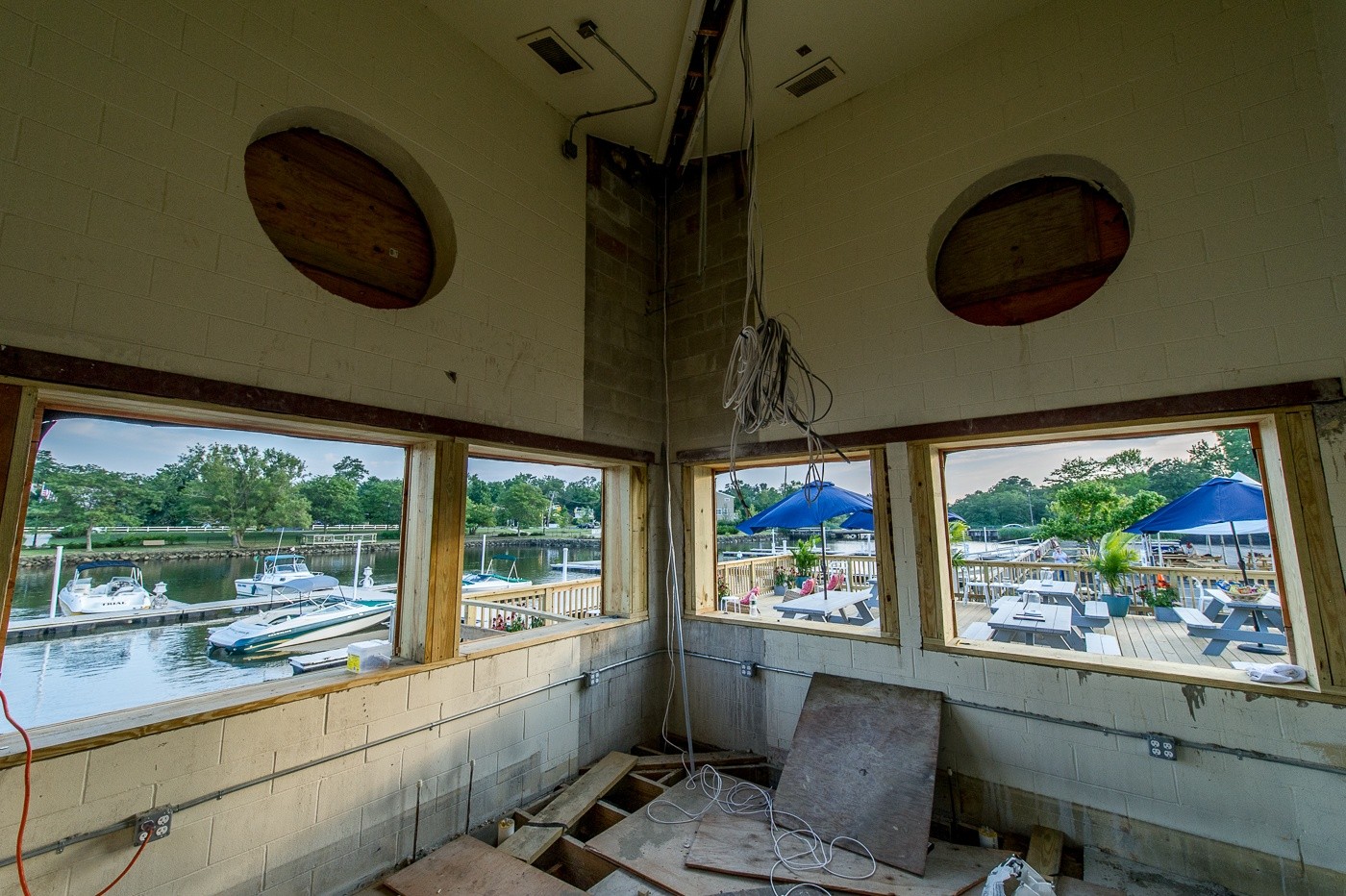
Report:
[1134,573,1183,610]
[717,573,731,604]
[773,565,799,589]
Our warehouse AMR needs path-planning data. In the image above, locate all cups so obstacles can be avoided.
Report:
[979,829,998,849]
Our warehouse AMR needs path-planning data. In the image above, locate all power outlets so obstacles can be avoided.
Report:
[581,669,601,689]
[131,802,175,848]
[1146,733,1179,763]
[740,660,759,678]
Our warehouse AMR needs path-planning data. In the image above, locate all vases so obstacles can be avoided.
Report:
[718,599,729,611]
[1152,605,1182,623]
[773,582,790,597]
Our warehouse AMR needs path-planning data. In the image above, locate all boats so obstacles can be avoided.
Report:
[57,558,169,616]
[461,534,532,588]
[233,526,324,596]
[205,540,398,659]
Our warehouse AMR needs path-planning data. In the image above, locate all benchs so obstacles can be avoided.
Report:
[793,613,809,620]
[990,595,1022,611]
[1083,600,1111,620]
[1083,632,1121,656]
[861,617,880,630]
[961,620,996,643]
[1156,561,1284,630]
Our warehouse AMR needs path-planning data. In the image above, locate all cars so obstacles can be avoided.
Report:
[1001,524,1023,528]
[576,523,595,529]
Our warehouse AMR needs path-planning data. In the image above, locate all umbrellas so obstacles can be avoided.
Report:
[840,503,967,530]
[1123,470,1285,654]
[736,479,872,600]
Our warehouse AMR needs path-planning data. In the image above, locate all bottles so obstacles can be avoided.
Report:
[749,593,757,619]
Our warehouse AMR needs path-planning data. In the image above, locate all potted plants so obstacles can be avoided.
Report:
[789,533,824,590]
[1075,526,1144,618]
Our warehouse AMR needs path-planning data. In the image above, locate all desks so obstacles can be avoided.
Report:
[987,599,1088,653]
[855,576,879,618]
[1243,555,1273,572]
[772,589,875,627]
[1185,563,1234,586]
[1183,559,1219,582]
[1015,579,1095,635]
[1184,587,1289,656]
